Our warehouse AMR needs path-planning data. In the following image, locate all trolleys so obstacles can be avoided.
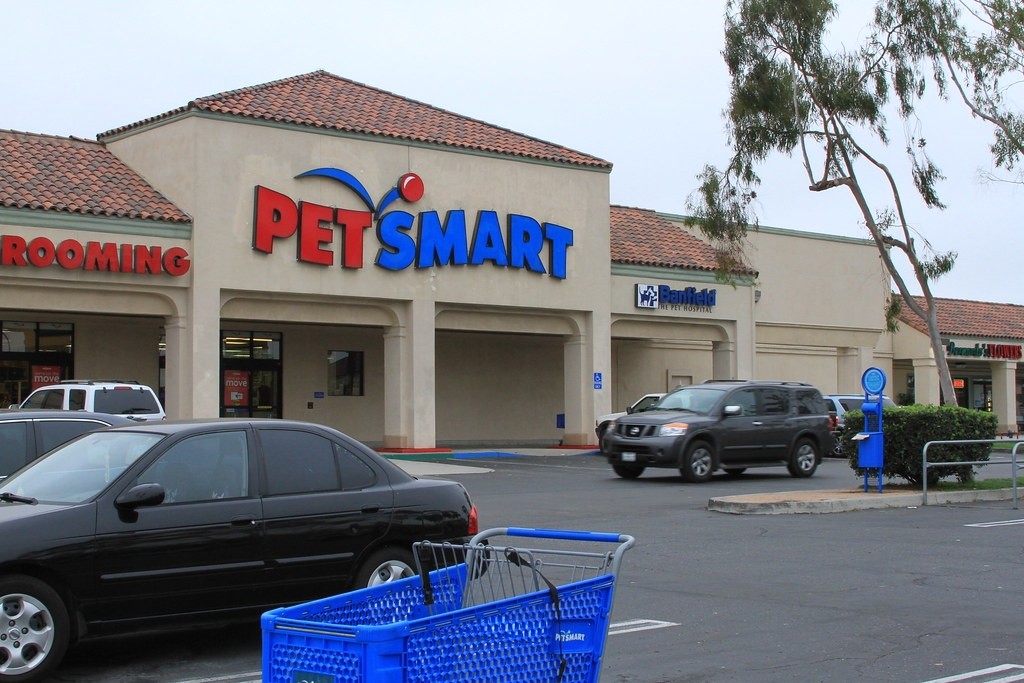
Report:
[262,527,634,683]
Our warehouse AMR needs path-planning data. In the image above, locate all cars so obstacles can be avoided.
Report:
[0,419,491,683]
[0,408,138,481]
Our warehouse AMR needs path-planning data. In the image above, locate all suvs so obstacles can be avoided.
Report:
[601,379,835,484]
[821,393,896,457]
[10,379,167,423]
[594,392,693,455]
